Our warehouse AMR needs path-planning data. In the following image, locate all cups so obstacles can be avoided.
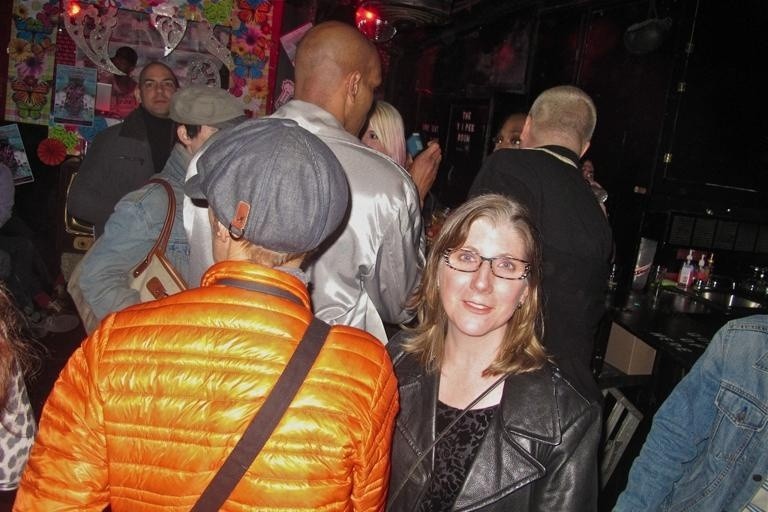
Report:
[631,238,658,291]
[424,202,453,249]
[649,262,669,290]
[606,264,623,293]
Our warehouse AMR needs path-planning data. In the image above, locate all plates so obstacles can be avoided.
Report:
[43,314,80,334]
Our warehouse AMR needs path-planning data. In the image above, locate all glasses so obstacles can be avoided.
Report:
[438,246,534,283]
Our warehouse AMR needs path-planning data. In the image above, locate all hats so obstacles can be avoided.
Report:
[167,83,248,127]
[181,115,352,254]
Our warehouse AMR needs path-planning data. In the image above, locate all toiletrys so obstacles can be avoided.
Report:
[706,252,716,278]
[688,254,708,287]
[677,249,695,288]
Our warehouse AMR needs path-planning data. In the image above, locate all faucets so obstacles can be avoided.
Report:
[696,280,720,295]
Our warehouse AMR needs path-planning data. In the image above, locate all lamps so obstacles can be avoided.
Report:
[353,2,397,42]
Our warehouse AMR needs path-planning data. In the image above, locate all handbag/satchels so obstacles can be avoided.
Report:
[126,176,193,307]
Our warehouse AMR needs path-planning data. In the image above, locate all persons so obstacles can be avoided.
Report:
[611,315,768,512]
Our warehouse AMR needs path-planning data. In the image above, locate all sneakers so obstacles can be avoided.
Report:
[52,280,71,307]
[25,308,83,334]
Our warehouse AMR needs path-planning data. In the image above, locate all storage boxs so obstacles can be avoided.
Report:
[603,322,657,377]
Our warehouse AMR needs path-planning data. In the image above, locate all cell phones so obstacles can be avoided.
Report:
[405,130,427,161]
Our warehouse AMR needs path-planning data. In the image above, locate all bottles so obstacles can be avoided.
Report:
[678,250,716,287]
[588,177,608,205]
[455,109,475,153]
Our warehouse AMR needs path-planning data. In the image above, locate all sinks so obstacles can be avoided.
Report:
[700,290,763,308]
